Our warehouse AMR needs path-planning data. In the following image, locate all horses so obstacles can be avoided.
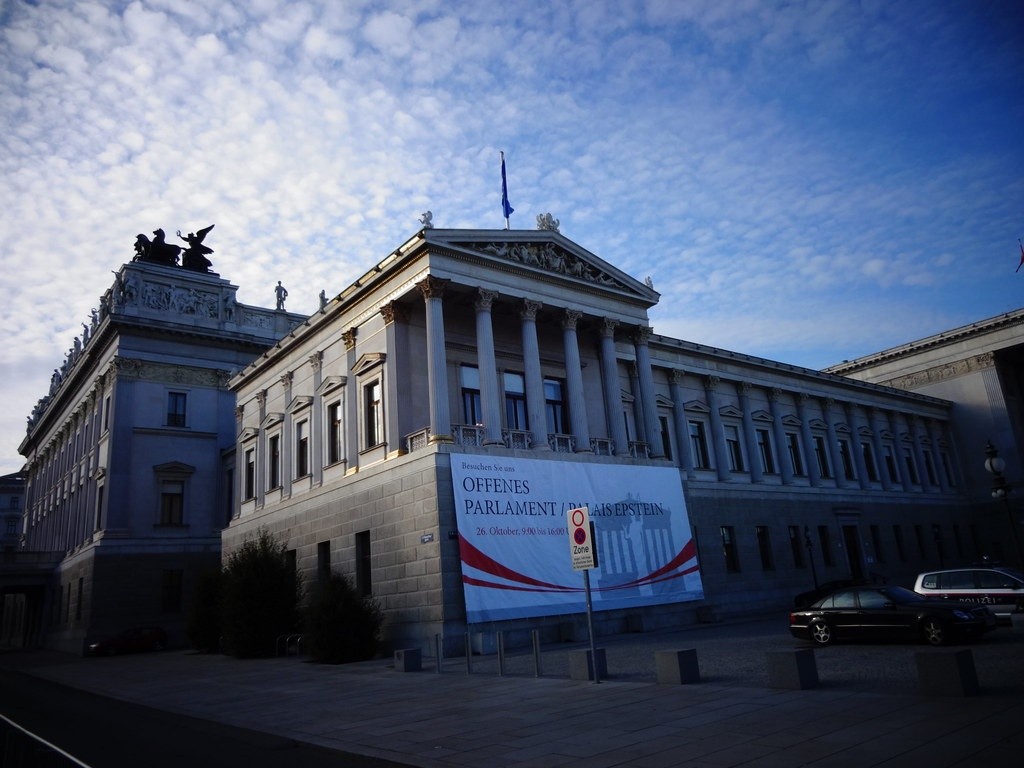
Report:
[133,228,188,268]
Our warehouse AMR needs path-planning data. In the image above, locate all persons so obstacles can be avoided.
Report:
[478,242,623,288]
[273,281,288,309]
[27,310,97,430]
[318,289,329,308]
[178,231,213,255]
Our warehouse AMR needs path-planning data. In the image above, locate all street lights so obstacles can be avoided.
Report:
[983,439,1018,568]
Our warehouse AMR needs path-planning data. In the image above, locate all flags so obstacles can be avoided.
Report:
[500,159,514,218]
[1016,244,1024,273]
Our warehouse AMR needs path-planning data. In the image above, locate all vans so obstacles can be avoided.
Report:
[915,569,1024,614]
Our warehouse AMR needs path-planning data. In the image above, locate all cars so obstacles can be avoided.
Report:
[789,586,998,648]
